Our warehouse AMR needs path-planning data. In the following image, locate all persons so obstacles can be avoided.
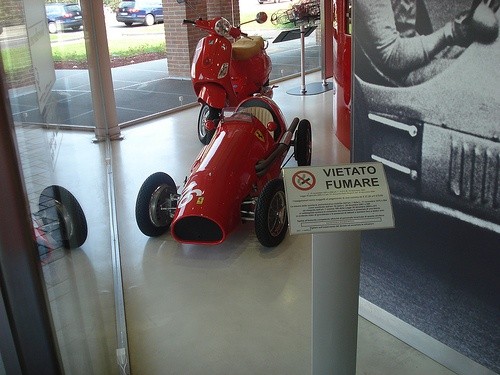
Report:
[353,0,499,86]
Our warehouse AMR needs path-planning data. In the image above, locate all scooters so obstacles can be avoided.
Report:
[181,11,279,144]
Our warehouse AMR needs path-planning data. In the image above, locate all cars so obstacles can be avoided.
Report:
[115,0,164,27]
[45,2,83,33]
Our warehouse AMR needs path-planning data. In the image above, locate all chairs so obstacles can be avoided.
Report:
[235,97,282,142]
